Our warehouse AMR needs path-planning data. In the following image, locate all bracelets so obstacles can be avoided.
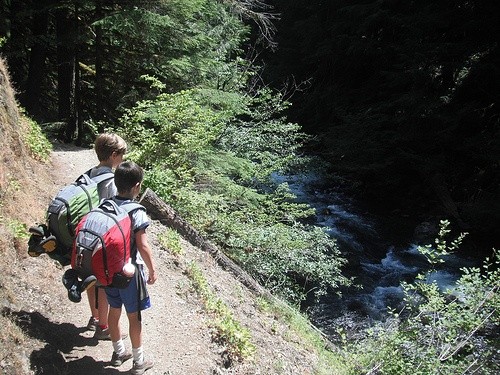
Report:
[149,270,155,275]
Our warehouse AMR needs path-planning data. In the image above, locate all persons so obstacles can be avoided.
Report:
[75,133,128,341]
[99,160,158,375]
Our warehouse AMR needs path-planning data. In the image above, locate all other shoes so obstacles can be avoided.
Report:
[26,223,59,258]
[61,268,97,304]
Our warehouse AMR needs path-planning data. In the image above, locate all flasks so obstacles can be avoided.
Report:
[123,264,135,283]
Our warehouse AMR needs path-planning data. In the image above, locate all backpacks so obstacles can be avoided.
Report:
[45,174,99,268]
[71,200,147,288]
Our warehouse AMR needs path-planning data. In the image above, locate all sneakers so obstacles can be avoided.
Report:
[132,358,156,375]
[111,348,133,366]
[87,315,128,340]
